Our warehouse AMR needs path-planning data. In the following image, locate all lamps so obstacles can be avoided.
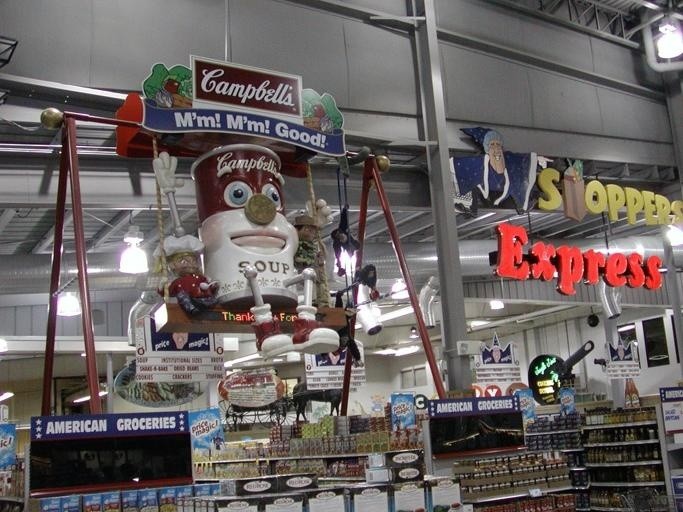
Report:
[354,303,382,337]
[598,274,623,321]
[413,275,442,332]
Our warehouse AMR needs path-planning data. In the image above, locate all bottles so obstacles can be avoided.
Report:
[583,410,669,508]
